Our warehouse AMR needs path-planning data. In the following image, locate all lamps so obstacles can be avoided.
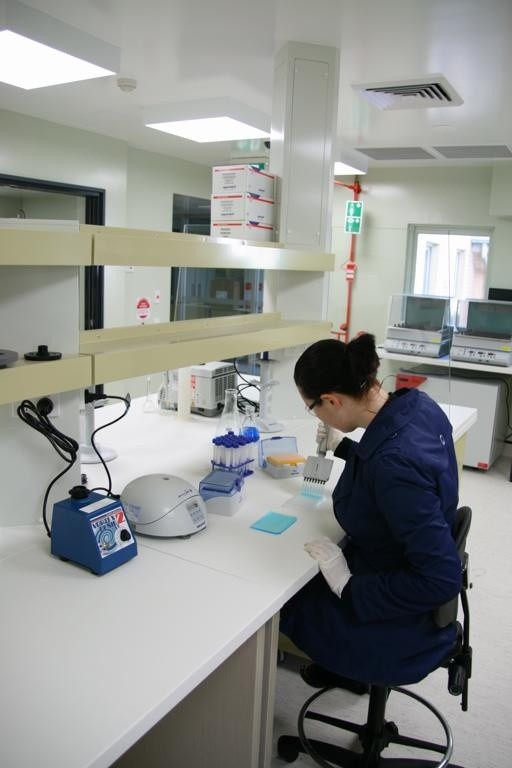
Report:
[0,0,122,90]
[142,96,270,142]
[334,142,369,176]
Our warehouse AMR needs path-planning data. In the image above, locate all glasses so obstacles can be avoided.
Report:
[302,396,323,418]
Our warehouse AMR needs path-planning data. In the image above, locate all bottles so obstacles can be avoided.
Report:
[210,384,262,482]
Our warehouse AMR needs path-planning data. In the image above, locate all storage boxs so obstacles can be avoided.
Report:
[211,165,275,243]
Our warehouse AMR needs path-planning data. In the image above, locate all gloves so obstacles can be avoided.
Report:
[314,421,346,453]
[302,535,353,601]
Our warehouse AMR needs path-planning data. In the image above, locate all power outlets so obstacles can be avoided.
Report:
[11,395,60,416]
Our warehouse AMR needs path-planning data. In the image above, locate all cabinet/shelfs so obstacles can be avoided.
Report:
[0,223,336,405]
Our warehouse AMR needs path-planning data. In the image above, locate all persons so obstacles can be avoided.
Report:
[276,333,461,687]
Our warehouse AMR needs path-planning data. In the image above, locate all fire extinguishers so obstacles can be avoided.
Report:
[245,283,251,303]
